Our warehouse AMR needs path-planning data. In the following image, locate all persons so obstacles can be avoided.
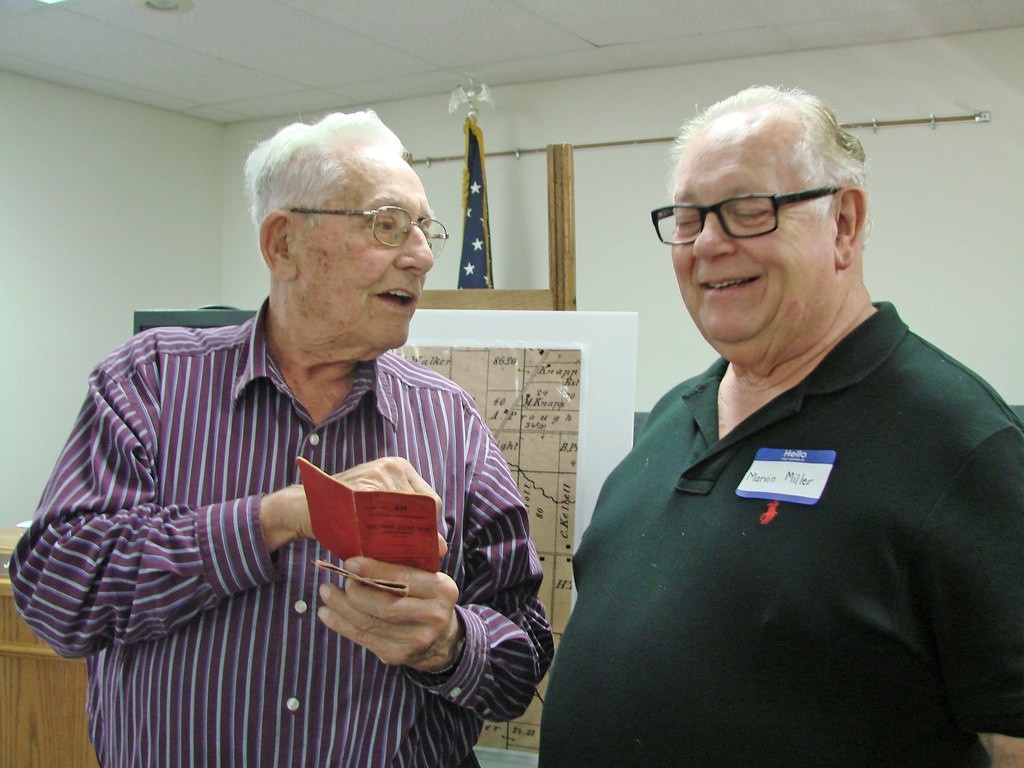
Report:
[9,108,552,768]
[537,87,1024,768]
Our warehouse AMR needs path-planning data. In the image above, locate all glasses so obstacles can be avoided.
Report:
[290,206,449,259]
[651,186,844,246]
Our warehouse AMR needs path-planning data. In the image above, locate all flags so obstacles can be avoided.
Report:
[456,116,494,289]
